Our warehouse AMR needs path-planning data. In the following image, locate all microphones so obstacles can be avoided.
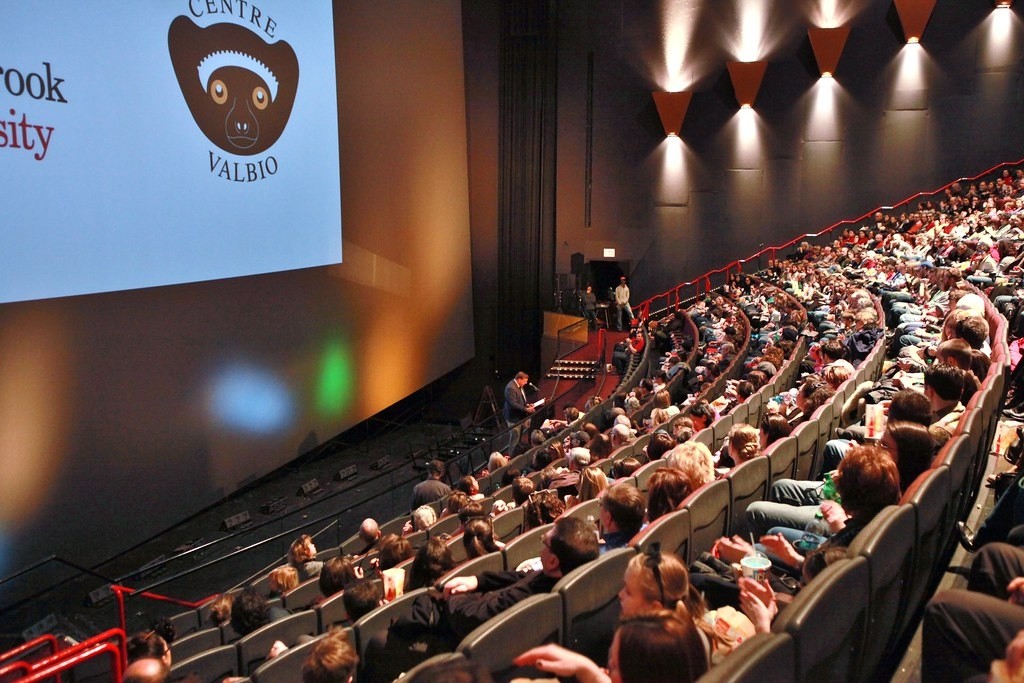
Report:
[529,383,539,391]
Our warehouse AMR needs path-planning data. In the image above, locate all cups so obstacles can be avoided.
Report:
[383,568,405,602]
[740,550,772,586]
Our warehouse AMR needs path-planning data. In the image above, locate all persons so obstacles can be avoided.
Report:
[121,165,1024,683]
[582,281,600,331]
[614,276,635,331]
[503,372,536,459]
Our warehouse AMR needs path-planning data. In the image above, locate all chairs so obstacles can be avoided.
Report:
[165,271,1024,683]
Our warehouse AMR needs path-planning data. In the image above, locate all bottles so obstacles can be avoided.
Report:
[770,391,798,406]
[930,331,940,346]
[924,359,932,380]
[823,473,841,505]
[495,483,501,492]
[799,512,824,551]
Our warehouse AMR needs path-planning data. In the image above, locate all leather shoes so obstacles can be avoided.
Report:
[956,520,977,553]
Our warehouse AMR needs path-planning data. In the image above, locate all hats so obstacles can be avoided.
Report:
[621,277,626,280]
[752,361,777,376]
[587,287,592,293]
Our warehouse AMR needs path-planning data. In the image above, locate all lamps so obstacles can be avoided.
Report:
[807,25,851,77]
[995,0,1013,8]
[894,0,937,44]
[652,90,694,136]
[726,60,768,108]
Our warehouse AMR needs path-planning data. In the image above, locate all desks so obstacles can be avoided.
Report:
[594,303,611,328]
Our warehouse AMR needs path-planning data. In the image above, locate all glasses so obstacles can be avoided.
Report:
[645,542,665,606]
[539,535,553,552]
[815,382,828,388]
[465,516,493,534]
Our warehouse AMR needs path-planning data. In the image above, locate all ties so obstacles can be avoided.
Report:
[519,387,526,405]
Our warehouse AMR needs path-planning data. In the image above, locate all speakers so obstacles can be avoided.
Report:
[571,252,584,274]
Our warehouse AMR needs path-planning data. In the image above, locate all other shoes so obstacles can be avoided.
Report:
[835,428,844,439]
[1002,408,1024,421]
[987,474,1000,485]
[619,328,623,332]
